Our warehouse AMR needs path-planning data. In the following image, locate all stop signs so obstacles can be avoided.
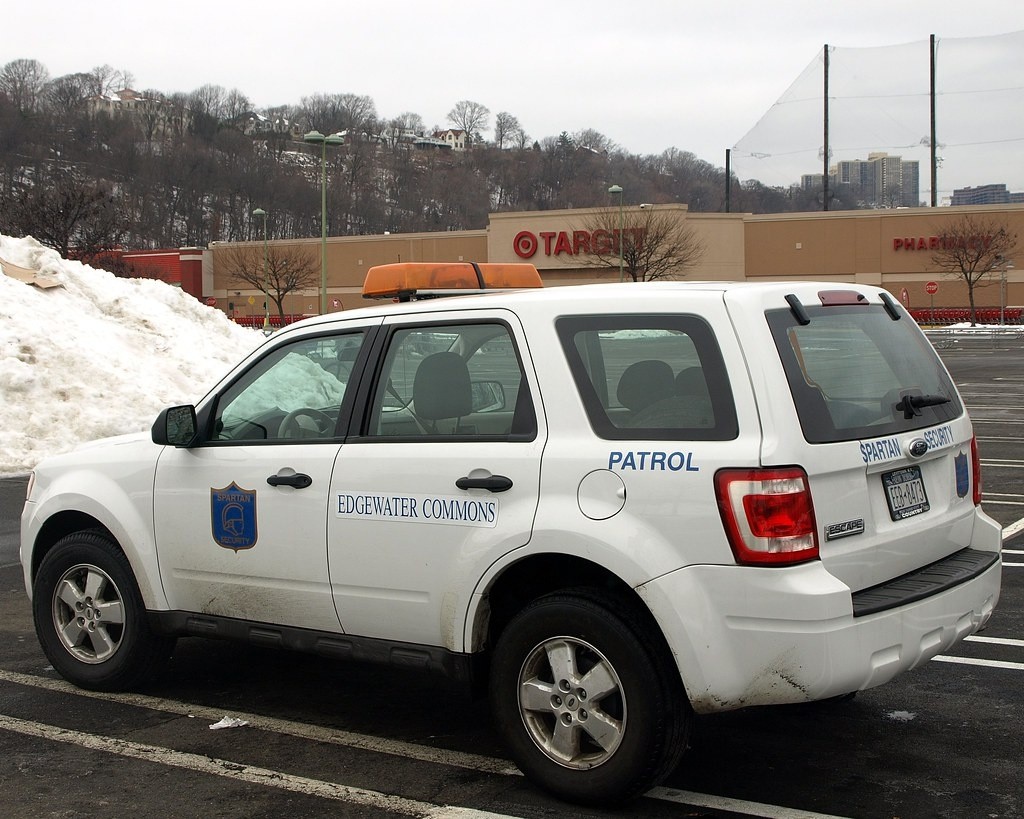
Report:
[206,297,217,307]
[925,281,939,295]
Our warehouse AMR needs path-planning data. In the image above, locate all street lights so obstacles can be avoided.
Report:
[304,133,344,315]
[252,208,270,328]
[608,184,623,282]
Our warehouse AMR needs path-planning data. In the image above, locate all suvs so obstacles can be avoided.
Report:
[17,262,1003,810]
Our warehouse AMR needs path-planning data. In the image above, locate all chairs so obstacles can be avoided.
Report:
[413,351,472,434]
[616,360,676,429]
